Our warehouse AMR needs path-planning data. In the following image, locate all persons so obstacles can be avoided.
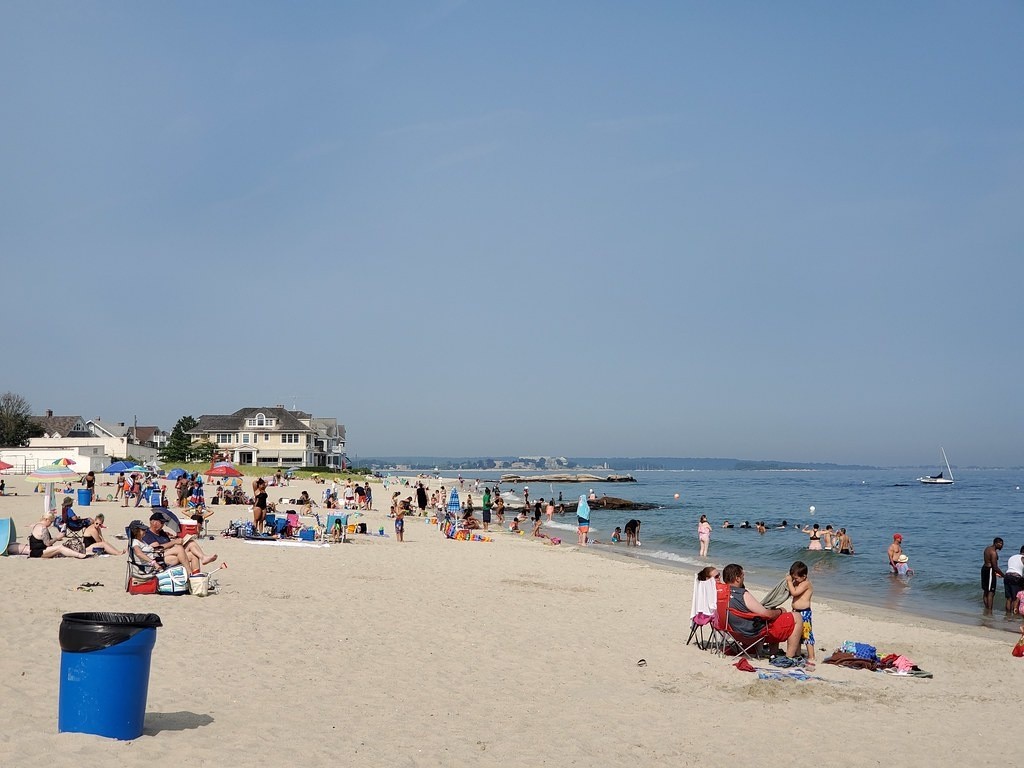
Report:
[0,468,643,580]
[721,518,856,556]
[980,537,1024,616]
[697,514,713,556]
[698,561,817,672]
[888,532,914,578]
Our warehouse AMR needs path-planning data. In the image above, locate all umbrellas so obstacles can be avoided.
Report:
[311,473,320,477]
[446,486,461,531]
[52,458,76,469]
[150,507,182,534]
[0,459,14,471]
[23,465,83,516]
[187,476,206,510]
[432,466,440,474]
[222,476,243,486]
[286,466,301,476]
[121,465,154,473]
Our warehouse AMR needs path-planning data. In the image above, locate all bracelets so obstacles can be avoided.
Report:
[777,607,783,616]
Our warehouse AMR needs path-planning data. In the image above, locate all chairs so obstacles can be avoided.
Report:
[686,573,742,657]
[55,515,83,538]
[188,509,205,538]
[707,580,770,660]
[264,514,291,537]
[124,527,172,592]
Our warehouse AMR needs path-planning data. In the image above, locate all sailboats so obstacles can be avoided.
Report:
[920,447,955,484]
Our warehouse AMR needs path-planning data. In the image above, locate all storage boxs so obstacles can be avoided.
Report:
[177,519,198,539]
[327,514,349,534]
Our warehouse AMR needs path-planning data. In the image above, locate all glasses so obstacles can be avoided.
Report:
[707,572,720,579]
[95,523,102,526]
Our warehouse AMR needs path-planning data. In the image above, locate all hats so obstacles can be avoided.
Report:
[150,512,169,523]
[897,555,909,563]
[129,519,148,530]
[42,511,55,522]
[61,496,74,506]
[893,533,903,539]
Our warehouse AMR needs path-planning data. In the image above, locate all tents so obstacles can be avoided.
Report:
[204,465,245,478]
[102,461,141,474]
[166,468,190,481]
[144,459,168,466]
[214,462,235,468]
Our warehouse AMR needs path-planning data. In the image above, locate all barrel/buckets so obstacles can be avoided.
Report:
[77,489,92,506]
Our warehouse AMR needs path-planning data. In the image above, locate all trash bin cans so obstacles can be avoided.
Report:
[78,489,92,506]
[58,612,163,740]
[147,486,159,503]
